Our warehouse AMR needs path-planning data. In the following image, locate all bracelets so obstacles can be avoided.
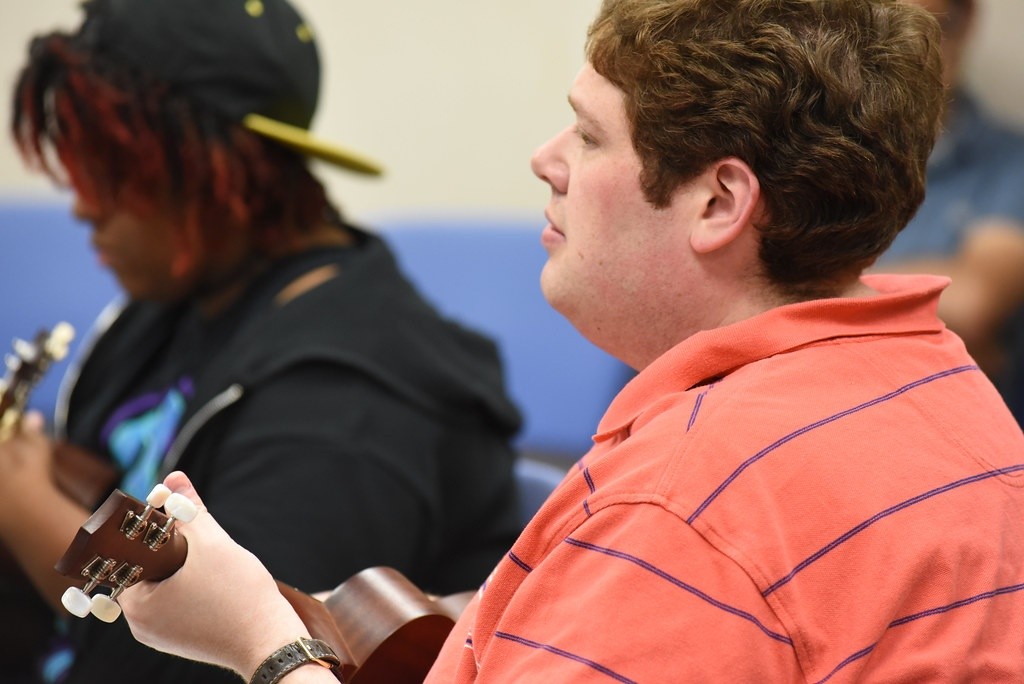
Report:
[249,637,339,684]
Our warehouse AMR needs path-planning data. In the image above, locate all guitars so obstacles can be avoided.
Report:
[0,320,123,513]
[53,488,477,684]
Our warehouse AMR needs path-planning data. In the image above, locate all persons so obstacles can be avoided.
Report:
[0,0,526,684]
[106,2,1024,684]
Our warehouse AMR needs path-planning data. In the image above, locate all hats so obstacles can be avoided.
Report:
[79,0,379,174]
